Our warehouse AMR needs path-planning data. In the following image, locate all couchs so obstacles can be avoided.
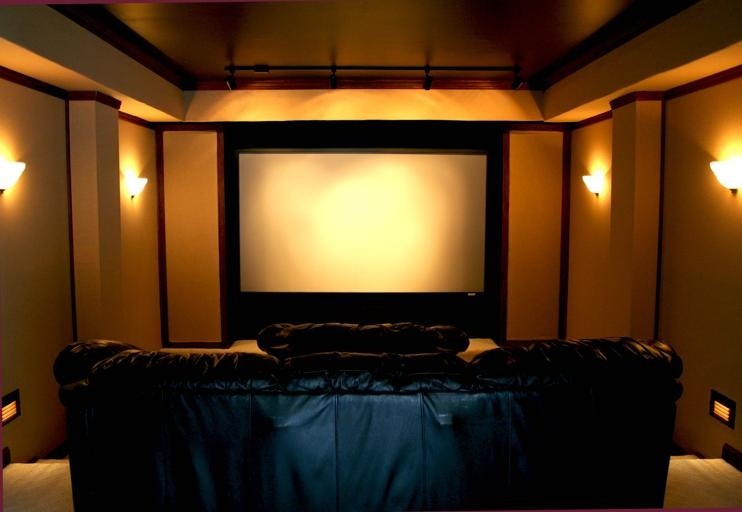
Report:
[51,318,684,511]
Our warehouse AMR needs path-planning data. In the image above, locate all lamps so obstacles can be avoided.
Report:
[581,171,606,197]
[706,156,742,195]
[125,176,149,199]
[0,160,26,196]
